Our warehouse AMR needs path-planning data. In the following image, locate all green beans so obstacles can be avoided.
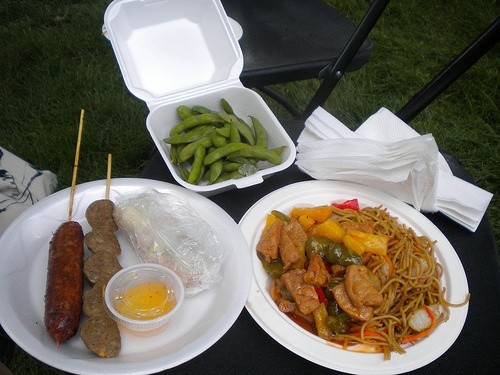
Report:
[162,98,285,184]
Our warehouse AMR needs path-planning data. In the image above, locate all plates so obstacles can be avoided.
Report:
[1,177,253,375]
[238,180,470,375]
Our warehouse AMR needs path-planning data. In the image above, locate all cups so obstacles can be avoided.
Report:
[105,264,185,331]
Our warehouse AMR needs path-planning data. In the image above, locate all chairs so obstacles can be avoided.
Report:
[220,0,391,119]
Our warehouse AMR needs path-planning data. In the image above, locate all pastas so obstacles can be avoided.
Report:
[255,198,470,360]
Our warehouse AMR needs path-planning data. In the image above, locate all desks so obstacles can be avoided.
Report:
[57,16,500,375]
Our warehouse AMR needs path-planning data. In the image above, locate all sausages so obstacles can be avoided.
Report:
[44,220,83,344]
